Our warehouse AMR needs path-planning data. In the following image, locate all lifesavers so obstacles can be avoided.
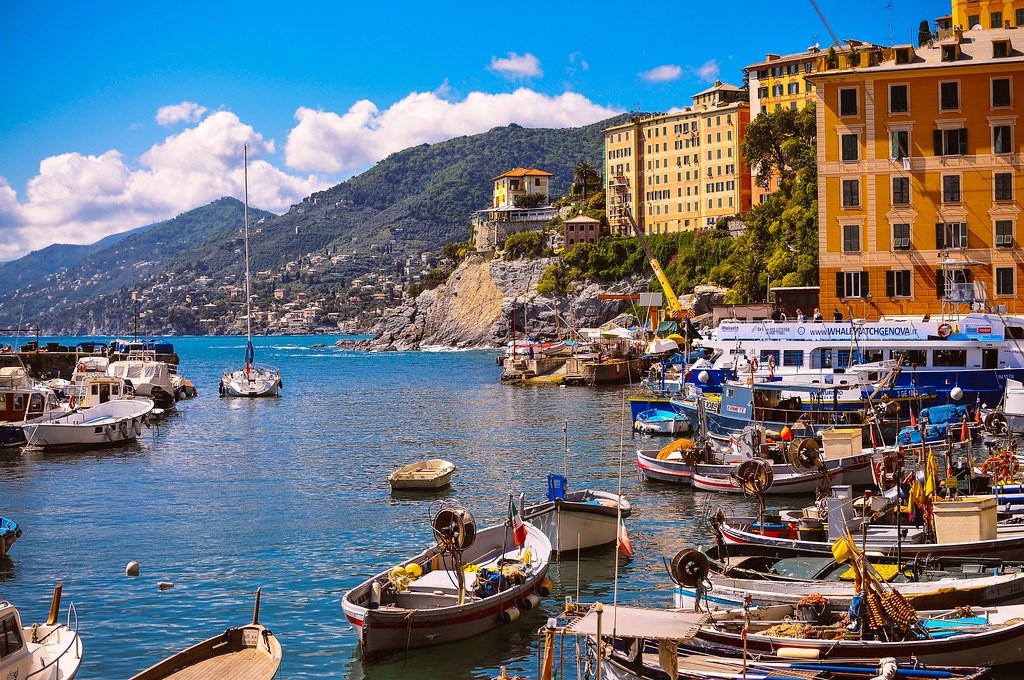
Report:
[752,358,758,371]
[997,453,1019,476]
[77,363,85,371]
[768,355,775,368]
[938,323,952,338]
[982,457,1007,481]
[876,455,905,484]
[70,395,75,409]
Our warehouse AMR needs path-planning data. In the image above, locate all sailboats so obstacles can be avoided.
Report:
[506,294,587,361]
[220,140,281,397]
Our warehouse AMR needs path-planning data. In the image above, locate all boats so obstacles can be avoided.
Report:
[649,313,1024,496]
[0,298,195,446]
[0,517,84,680]
[542,497,1024,680]
[341,417,635,667]
[126,586,283,680]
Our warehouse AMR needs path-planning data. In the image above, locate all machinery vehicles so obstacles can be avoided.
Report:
[617,204,705,353]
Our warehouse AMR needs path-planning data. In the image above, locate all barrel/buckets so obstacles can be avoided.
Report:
[796,602,831,626]
[787,521,797,539]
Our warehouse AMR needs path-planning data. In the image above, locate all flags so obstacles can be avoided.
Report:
[508,500,527,548]
[618,516,633,556]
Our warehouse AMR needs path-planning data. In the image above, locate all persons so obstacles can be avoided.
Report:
[780,312,786,323]
[796,309,804,323]
[833,308,845,323]
[813,308,824,323]
[771,306,780,323]
